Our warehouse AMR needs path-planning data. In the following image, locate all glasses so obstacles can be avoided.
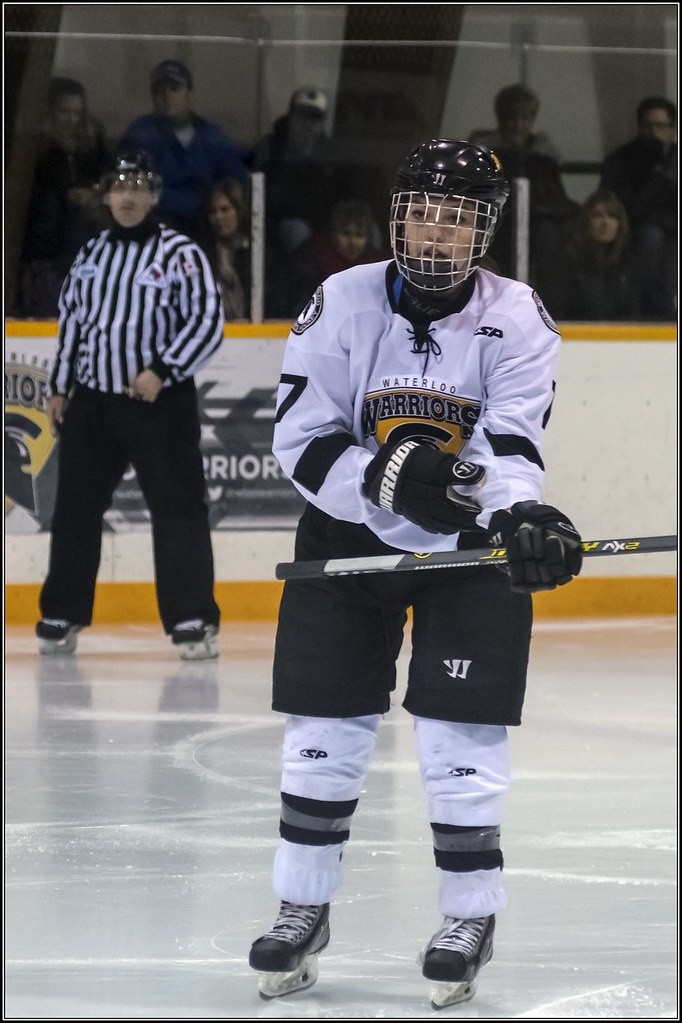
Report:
[152,83,179,95]
[642,121,669,129]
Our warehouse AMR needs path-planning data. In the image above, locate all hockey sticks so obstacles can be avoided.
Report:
[276,533,678,583]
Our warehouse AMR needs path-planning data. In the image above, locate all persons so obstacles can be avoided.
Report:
[247,137,584,1014]
[19,59,678,323]
[35,150,223,660]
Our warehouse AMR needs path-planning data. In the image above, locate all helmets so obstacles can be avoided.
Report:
[101,146,164,200]
[389,137,511,291]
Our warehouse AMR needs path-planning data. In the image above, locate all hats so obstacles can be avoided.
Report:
[151,60,192,86]
[290,86,329,117]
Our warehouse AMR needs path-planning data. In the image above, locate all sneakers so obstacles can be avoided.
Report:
[35,618,83,654]
[249,901,331,997]
[172,614,219,660]
[422,915,496,1011]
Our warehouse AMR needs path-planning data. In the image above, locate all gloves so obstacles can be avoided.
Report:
[485,500,582,592]
[361,440,486,535]
